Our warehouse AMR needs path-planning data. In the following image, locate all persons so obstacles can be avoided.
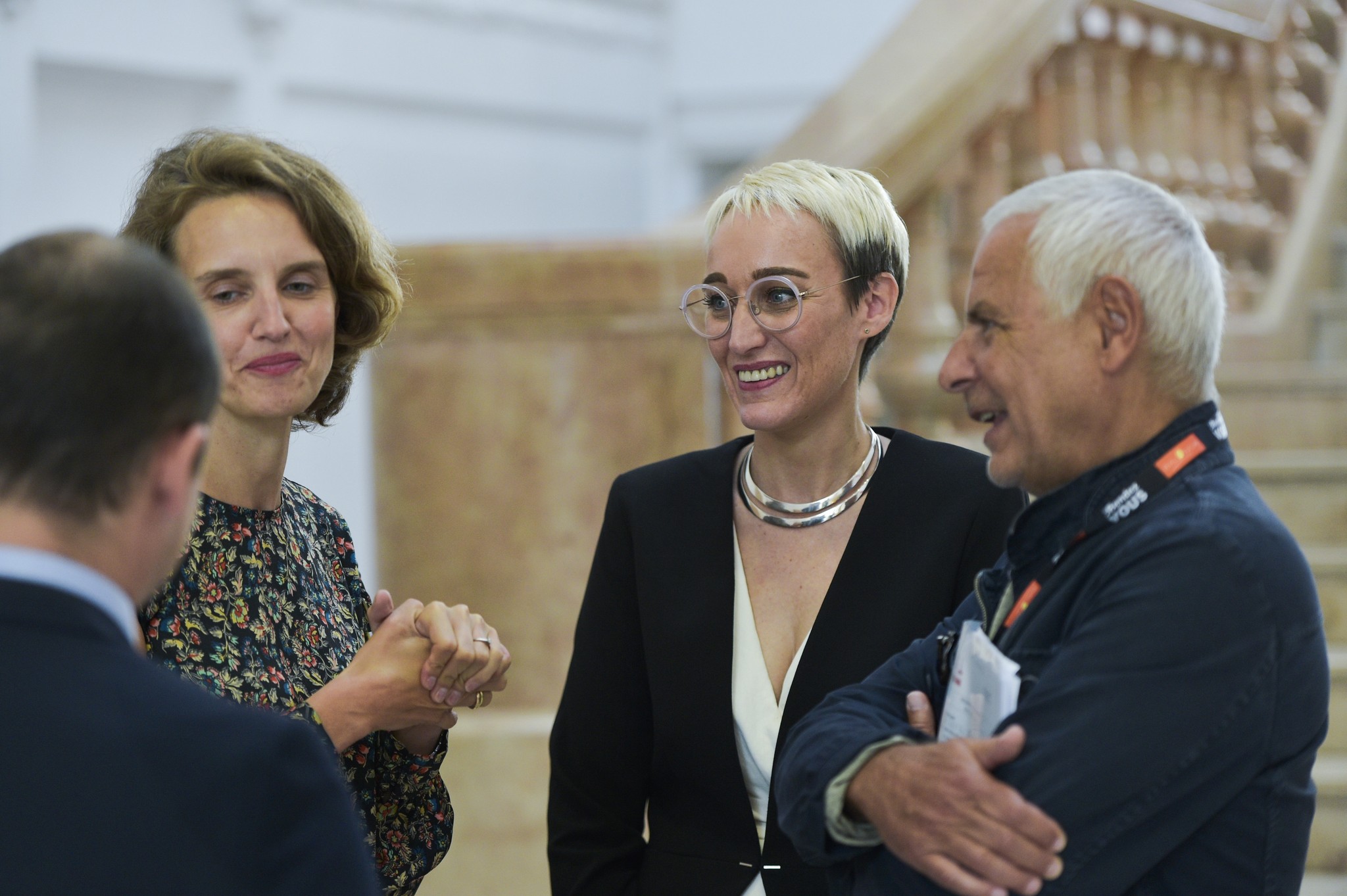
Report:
[0,230,381,895]
[774,166,1329,895]
[115,130,509,896]
[545,158,1033,895]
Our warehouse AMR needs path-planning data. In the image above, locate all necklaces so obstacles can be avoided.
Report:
[736,421,884,528]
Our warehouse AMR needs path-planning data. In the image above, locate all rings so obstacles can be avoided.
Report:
[468,691,484,710]
[473,638,490,649]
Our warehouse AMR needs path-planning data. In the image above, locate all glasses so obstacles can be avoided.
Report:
[678,274,860,340]
